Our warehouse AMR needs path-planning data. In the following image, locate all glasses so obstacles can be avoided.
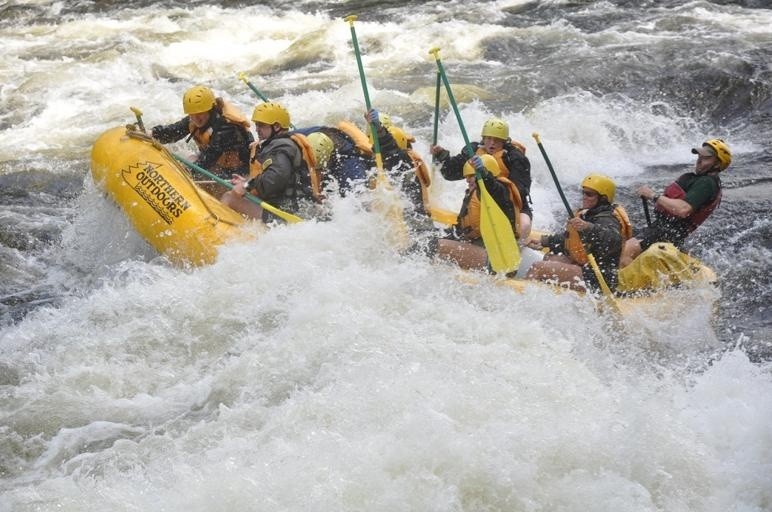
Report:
[581,191,597,198]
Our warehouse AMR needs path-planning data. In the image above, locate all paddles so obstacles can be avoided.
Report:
[533,132,625,317]
[428,47,521,273]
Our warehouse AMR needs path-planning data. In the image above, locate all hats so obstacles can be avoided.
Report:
[692,145,716,159]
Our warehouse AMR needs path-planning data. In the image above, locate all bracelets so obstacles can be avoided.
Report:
[651,192,661,204]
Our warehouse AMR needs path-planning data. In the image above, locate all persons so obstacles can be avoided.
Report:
[130,86,258,187]
[357,108,434,230]
[280,124,367,198]
[422,154,525,282]
[525,171,623,295]
[219,102,328,229]
[427,117,535,242]
[617,137,732,270]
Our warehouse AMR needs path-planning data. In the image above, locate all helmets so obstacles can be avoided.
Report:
[481,118,511,142]
[365,113,407,155]
[702,139,732,172]
[182,85,217,116]
[252,102,290,129]
[581,174,617,203]
[306,131,335,170]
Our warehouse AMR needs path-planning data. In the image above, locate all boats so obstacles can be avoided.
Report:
[88,122,723,337]
